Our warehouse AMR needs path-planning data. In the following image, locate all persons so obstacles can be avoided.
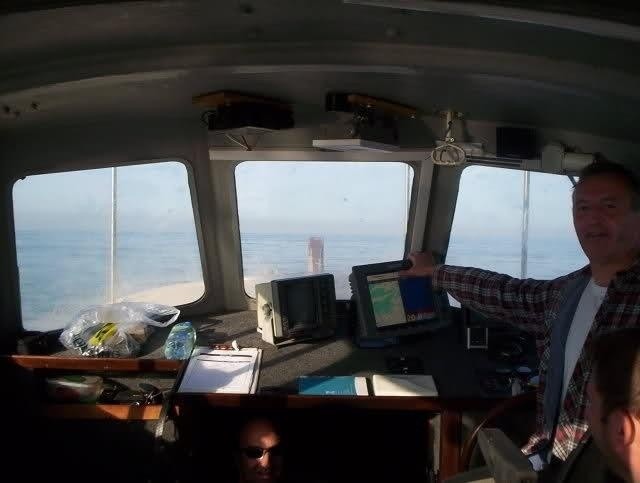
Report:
[580,329,640,483]
[234,416,285,482]
[397,160,639,466]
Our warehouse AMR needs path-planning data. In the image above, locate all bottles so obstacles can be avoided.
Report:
[163,321,196,361]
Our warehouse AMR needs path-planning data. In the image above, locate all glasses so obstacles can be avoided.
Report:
[239,445,270,460]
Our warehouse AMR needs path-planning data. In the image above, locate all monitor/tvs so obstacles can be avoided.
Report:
[255,274,337,349]
[496,126,536,159]
[348,258,453,341]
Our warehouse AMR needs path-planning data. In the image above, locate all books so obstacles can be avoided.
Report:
[311,138,399,151]
[372,373,440,397]
[298,374,369,396]
